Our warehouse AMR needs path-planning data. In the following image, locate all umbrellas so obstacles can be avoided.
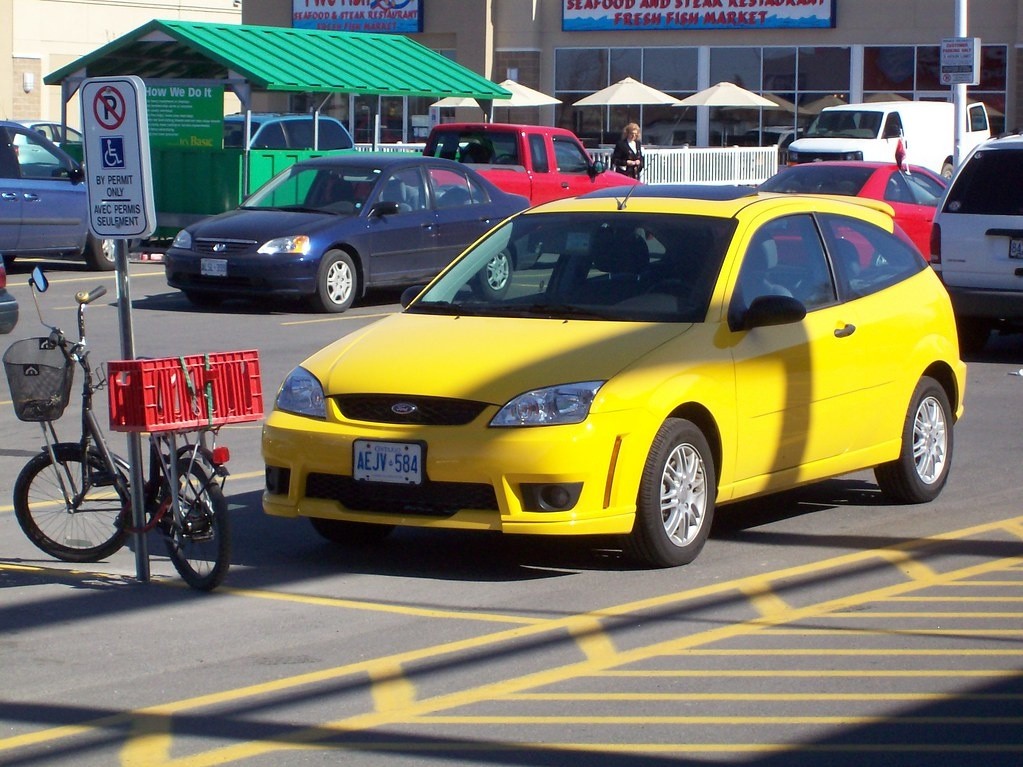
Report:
[722,93,911,115]
[966,97,1002,117]
[671,82,779,148]
[571,76,681,126]
[430,79,562,125]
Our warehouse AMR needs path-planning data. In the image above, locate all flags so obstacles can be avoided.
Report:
[895,137,907,171]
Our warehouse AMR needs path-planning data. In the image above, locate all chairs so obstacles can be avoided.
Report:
[460,143,492,164]
[439,188,478,207]
[809,238,863,303]
[709,232,793,317]
[837,180,857,195]
[382,179,412,213]
[576,226,664,312]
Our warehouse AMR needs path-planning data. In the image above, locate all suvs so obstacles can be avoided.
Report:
[927,129,1023,356]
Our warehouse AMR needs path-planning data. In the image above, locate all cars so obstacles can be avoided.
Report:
[8,120,84,172]
[0,248,20,334]
[162,153,534,315]
[0,120,119,270]
[717,161,951,271]
[261,184,968,569]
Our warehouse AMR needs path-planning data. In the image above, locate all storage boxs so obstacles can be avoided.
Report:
[106,348,264,432]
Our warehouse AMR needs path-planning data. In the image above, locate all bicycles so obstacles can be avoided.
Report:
[0,264,236,592]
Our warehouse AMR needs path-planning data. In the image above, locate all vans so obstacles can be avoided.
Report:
[222,112,356,152]
[786,101,991,185]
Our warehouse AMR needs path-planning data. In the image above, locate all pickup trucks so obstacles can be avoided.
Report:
[311,121,649,210]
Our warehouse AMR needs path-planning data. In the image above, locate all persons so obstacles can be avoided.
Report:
[612,122,645,181]
[733,249,794,311]
